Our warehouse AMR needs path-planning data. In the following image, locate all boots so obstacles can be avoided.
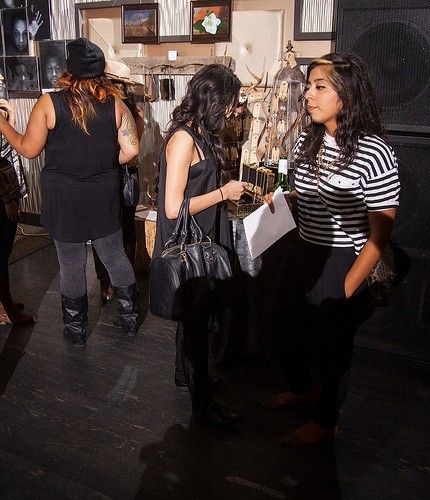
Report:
[59,292,89,352]
[108,282,148,337]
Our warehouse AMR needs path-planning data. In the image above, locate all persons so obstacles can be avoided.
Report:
[263,52,401,448]
[0,0,64,91]
[0,73,36,325]
[0,38,139,349]
[153,64,252,427]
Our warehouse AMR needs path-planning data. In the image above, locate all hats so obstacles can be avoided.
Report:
[104,60,135,85]
[65,38,105,78]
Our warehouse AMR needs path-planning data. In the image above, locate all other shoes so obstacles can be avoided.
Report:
[260,378,323,410]
[280,420,337,448]
[100,279,116,301]
[0,301,37,324]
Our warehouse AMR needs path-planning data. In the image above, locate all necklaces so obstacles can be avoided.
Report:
[320,143,343,170]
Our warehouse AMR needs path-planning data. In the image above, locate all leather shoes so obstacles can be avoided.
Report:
[192,402,242,427]
[174,369,223,393]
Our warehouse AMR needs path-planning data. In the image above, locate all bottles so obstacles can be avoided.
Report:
[273,157,291,192]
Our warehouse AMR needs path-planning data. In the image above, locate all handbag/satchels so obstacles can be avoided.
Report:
[365,238,398,305]
[148,197,241,320]
[121,163,139,206]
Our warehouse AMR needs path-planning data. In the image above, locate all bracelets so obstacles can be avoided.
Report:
[219,188,223,201]
